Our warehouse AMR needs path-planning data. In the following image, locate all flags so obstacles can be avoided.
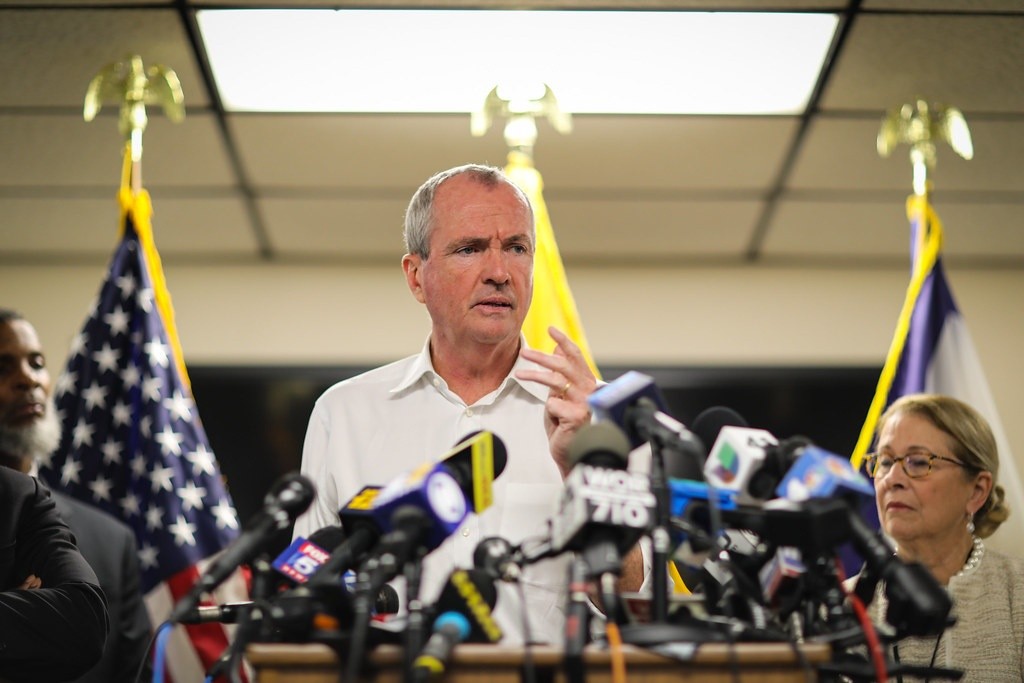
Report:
[32,184,252,683]
[850,199,1022,570]
[486,159,602,392]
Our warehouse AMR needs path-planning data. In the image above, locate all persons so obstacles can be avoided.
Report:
[2,306,150,683]
[862,393,1024,683]
[284,162,672,683]
[0,466,106,683]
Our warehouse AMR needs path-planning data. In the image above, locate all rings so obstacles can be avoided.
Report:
[561,380,572,397]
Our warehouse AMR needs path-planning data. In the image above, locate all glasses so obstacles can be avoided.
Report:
[864,451,976,480]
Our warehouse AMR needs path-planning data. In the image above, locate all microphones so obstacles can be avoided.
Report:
[160,367,952,681]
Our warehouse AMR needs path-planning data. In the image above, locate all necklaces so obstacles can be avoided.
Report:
[893,537,984,578]
